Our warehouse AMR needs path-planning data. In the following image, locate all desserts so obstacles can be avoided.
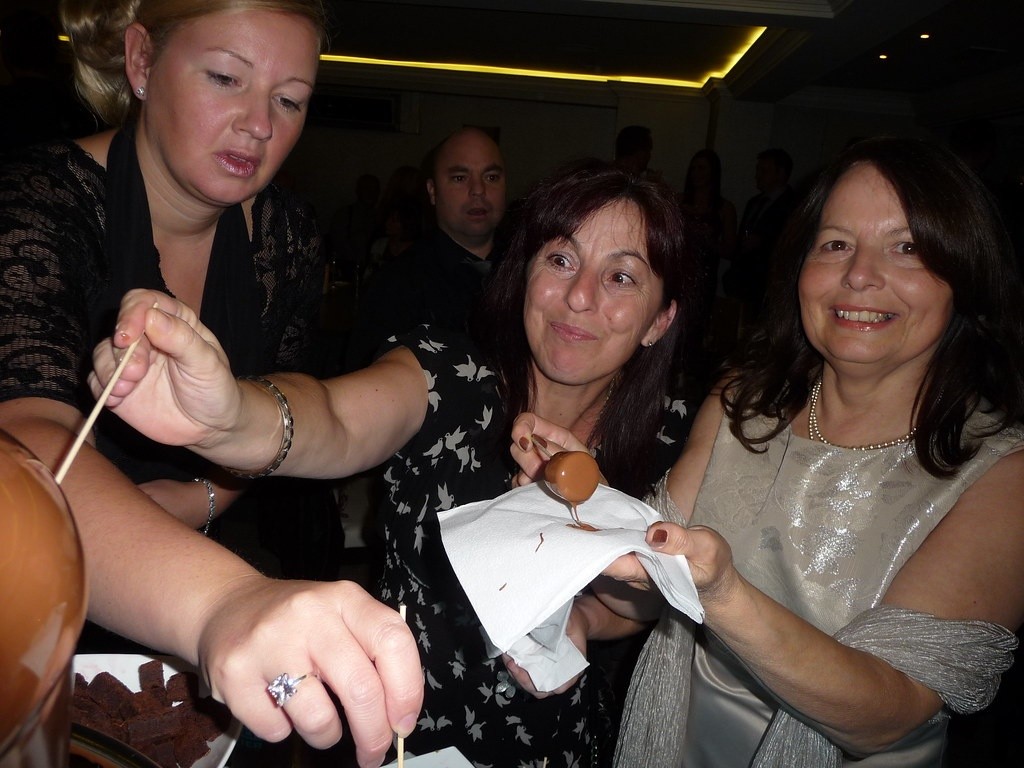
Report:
[542,450,599,506]
[0,421,86,768]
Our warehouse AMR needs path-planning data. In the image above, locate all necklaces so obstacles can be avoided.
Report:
[584,375,616,448]
[808,371,945,450]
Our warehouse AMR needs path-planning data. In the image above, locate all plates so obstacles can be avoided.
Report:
[73,653,242,768]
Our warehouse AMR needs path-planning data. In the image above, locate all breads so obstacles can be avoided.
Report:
[62,659,234,768]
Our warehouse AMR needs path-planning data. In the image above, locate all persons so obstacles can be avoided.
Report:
[508,136,1024,768]
[87,161,702,768]
[322,127,804,589]
[0,0,426,768]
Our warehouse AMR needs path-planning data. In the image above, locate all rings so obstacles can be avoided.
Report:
[266,664,318,706]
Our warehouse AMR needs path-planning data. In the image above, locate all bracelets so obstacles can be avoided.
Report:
[223,374,294,480]
[190,478,215,535]
